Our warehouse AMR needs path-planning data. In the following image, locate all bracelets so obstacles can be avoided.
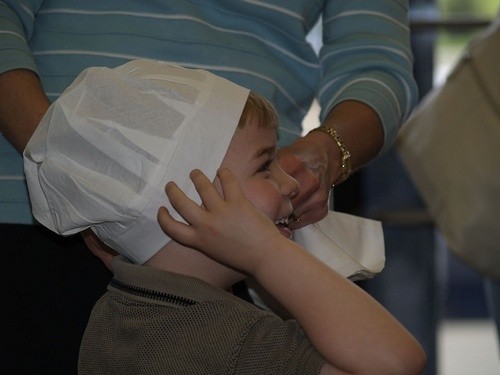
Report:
[308,124,352,187]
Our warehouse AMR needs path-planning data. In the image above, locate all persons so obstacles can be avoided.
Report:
[22,59,428,375]
[0,1,420,375]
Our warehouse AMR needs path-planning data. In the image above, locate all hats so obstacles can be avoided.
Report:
[23,59,252,266]
